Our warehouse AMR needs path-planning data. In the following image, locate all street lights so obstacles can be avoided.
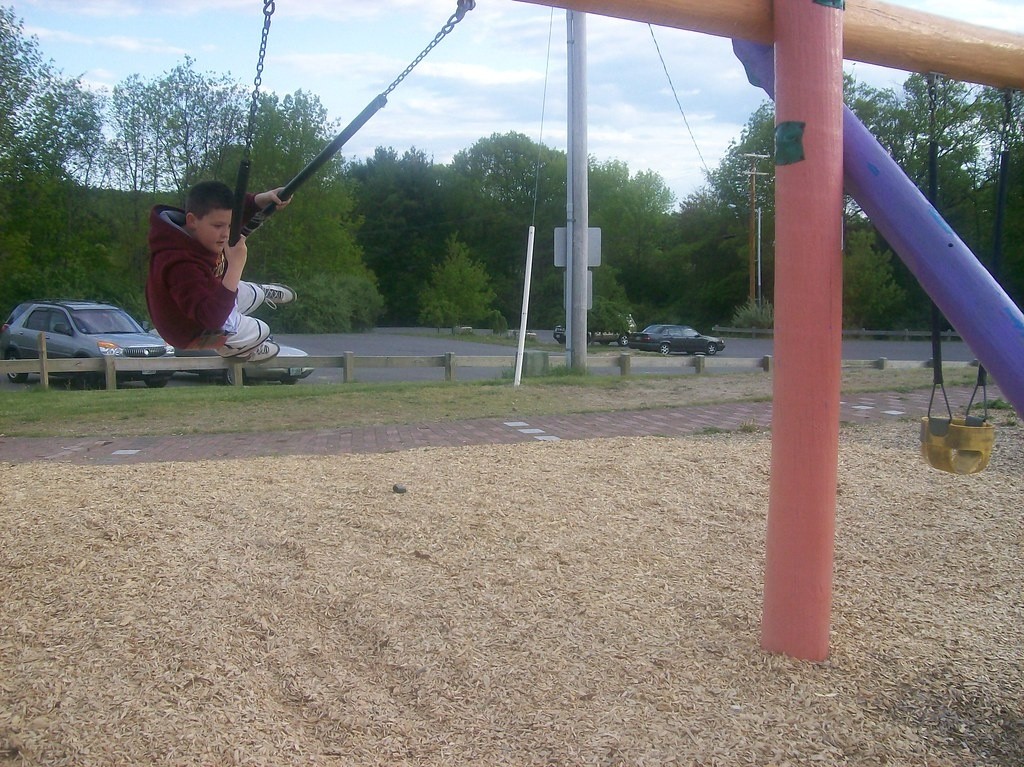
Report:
[726,203,761,310]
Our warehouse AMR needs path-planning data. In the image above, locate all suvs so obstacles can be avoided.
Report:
[552,315,637,347]
[0,297,176,391]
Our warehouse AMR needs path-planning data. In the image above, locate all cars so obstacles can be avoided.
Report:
[147,328,316,386]
[628,325,726,355]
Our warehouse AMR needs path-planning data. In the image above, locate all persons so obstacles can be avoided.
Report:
[145,181,298,363]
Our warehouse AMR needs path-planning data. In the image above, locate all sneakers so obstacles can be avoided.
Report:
[260,282,297,310]
[235,339,281,362]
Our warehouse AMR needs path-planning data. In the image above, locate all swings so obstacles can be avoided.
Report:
[919,68,1015,477]
[178,0,478,351]
